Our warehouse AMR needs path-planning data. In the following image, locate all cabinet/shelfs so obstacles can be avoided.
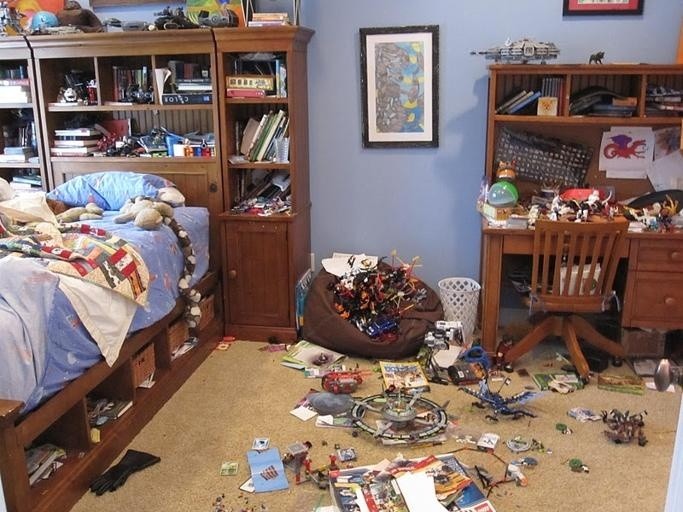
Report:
[213,26,308,341]
[26,29,223,333]
[478,58,682,361]
[1,35,49,196]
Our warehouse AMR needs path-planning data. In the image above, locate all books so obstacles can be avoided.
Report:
[49,128,102,157]
[225,60,287,99]
[113,60,212,104]
[0,77,32,104]
[89,392,135,420]
[22,447,60,485]
[1,121,42,189]
[492,76,638,119]
[234,169,291,203]
[251,11,292,24]
[235,109,290,162]
[245,20,293,28]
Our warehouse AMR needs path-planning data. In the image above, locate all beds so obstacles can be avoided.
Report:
[0,170,221,512]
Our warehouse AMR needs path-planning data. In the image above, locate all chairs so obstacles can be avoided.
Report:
[500,217,631,389]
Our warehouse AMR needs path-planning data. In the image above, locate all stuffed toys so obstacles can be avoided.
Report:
[163,213,202,339]
[46,198,104,224]
[112,198,174,229]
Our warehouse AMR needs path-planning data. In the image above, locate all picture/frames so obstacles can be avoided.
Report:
[359,24,440,151]
[561,0,645,17]
